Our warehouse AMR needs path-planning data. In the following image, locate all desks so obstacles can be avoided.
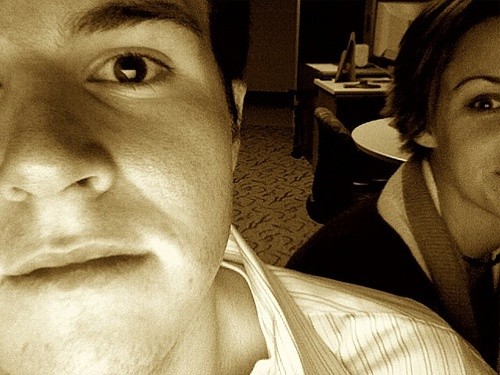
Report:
[307,62,385,75]
[352,117,412,161]
[316,79,392,94]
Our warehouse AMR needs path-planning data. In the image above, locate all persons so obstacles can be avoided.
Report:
[1,0,500,375]
[283,1,500,375]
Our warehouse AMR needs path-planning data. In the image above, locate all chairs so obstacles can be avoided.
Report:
[310,107,388,212]
